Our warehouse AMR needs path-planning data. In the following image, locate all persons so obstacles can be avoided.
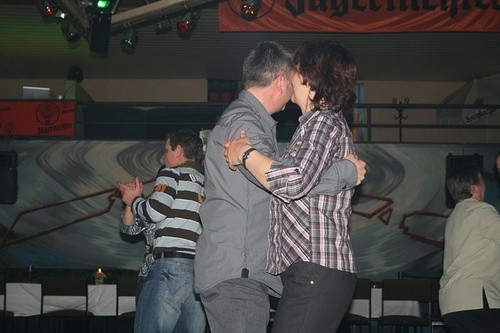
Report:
[118,41,367,333]
[438,153,500,333]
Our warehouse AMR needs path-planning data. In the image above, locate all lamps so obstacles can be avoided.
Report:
[155,6,202,40]
[225,0,276,21]
[35,0,137,59]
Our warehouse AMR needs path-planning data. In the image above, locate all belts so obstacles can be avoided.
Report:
[156,251,195,260]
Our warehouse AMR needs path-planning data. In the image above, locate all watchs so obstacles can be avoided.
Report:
[242,148,258,169]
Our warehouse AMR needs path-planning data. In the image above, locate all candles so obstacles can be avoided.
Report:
[96,267,103,284]
